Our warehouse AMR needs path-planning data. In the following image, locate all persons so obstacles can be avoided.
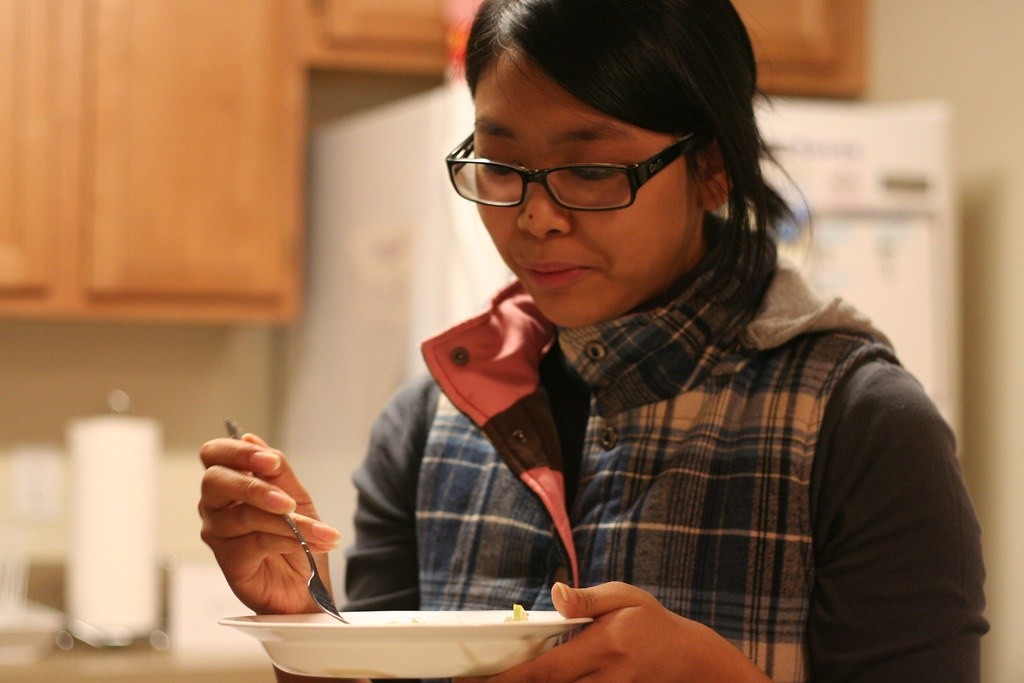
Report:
[197,0,987,682]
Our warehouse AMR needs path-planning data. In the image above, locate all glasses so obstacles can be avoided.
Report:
[447,129,704,212]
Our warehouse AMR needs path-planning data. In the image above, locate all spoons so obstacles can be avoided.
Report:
[223,420,347,625]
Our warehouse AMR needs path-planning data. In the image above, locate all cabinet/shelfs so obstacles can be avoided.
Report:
[0,0,871,326]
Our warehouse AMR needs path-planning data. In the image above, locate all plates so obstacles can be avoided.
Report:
[217,610,593,680]
